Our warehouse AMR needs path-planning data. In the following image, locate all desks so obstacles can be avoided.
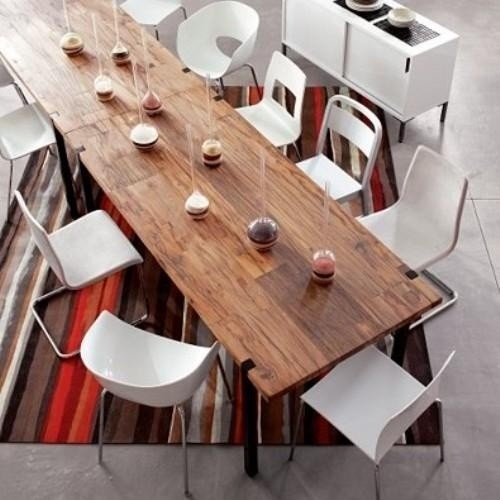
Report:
[64,84,443,477]
[281,0,460,142]
[0,0,204,220]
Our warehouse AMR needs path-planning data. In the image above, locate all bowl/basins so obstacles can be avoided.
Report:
[387,7,416,28]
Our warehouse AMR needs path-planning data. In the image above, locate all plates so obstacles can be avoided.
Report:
[345,0,384,12]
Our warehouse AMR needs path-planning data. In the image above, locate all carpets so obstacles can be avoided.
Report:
[0,86,443,446]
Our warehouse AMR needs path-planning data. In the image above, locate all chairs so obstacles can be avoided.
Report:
[0,102,57,224]
[233,51,309,148]
[287,344,457,500]
[80,310,234,495]
[358,145,468,330]
[176,0,260,100]
[12,189,151,360]
[295,94,382,201]
[119,0,188,41]
